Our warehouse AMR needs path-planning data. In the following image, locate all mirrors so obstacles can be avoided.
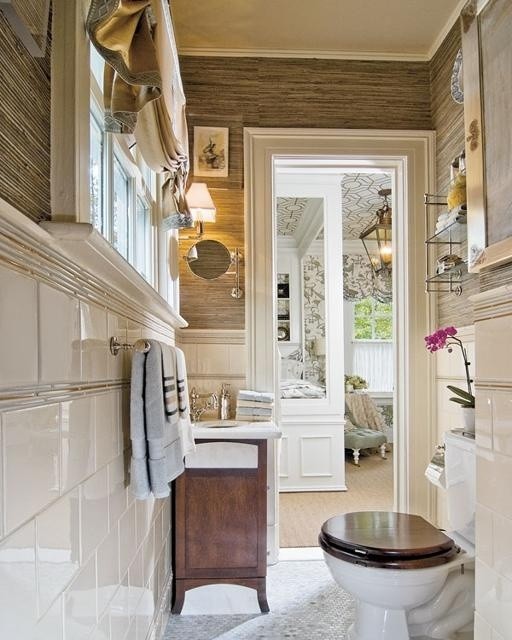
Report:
[183,240,242,299]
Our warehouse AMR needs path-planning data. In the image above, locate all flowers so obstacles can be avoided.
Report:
[426,325,476,407]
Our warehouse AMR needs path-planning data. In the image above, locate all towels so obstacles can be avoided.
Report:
[436,152,466,270]
[130,340,194,497]
[235,388,275,421]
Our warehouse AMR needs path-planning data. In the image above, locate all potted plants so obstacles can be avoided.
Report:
[345,374,368,395]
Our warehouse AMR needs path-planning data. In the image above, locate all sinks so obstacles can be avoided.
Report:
[200,420,238,429]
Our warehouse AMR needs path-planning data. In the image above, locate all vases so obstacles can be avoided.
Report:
[461,407,479,435]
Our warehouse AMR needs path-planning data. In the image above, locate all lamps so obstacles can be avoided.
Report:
[182,183,215,236]
[359,188,392,305]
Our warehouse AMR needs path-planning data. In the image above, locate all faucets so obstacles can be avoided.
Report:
[220,382,232,420]
[190,387,219,422]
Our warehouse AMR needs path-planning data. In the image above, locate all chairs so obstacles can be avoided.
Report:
[344,407,387,464]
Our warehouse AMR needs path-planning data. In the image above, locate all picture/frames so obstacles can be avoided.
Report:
[460,0,512,277]
[193,127,229,178]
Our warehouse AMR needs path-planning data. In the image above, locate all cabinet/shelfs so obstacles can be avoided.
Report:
[424,192,465,294]
[277,273,291,343]
[175,439,282,615]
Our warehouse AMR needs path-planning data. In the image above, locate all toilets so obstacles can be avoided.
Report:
[319,430,477,640]
